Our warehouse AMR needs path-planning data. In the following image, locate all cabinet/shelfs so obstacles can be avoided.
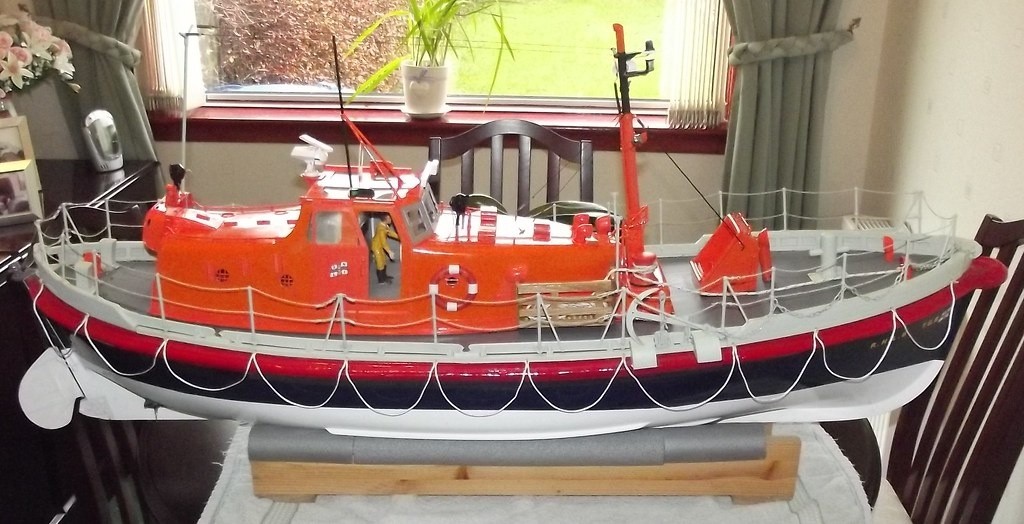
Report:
[1,158,160,524]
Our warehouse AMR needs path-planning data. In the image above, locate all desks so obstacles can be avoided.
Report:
[142,419,882,523]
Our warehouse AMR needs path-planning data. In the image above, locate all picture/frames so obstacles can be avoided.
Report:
[0,115,42,191]
[0,159,44,228]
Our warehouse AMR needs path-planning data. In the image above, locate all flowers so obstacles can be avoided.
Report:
[1,8,81,110]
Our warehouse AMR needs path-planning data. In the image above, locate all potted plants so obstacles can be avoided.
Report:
[343,0,516,119]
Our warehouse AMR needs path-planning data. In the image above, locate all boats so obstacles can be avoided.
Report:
[22,19,1011,445]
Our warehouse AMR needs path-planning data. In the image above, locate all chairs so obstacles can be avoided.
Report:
[427,118,592,220]
[870,213,1024,524]
[6,201,160,524]
